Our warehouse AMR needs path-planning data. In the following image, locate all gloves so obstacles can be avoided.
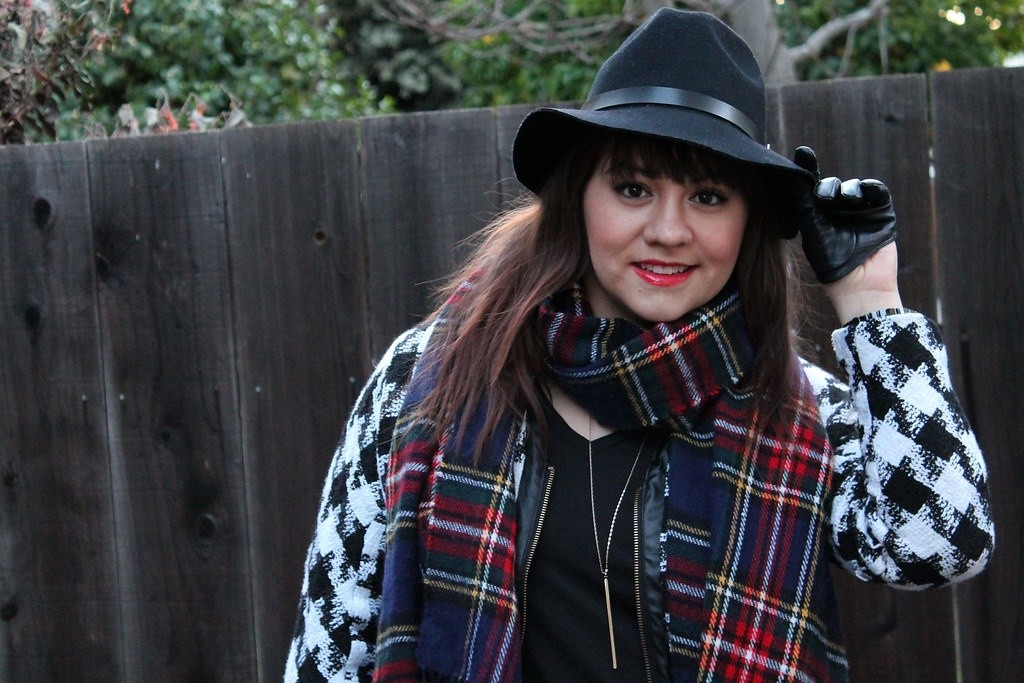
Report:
[791,144,900,285]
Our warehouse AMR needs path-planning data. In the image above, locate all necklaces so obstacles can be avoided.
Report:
[588,417,648,670]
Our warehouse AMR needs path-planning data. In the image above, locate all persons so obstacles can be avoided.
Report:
[283,7,996,683]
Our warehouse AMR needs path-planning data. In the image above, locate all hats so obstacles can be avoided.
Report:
[510,7,815,240]
